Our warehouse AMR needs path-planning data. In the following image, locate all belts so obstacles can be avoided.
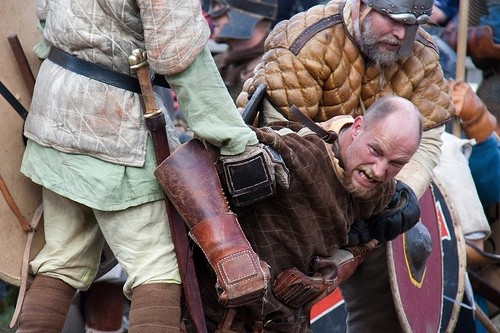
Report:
[48,44,142,94]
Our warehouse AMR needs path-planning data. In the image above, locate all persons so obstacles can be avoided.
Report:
[428,0,500,138]
[209,0,279,103]
[431,131,493,263]
[235,0,456,332]
[15,0,291,333]
[153,95,423,332]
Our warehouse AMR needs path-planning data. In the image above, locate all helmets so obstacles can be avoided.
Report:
[203,0,279,38]
[361,0,436,24]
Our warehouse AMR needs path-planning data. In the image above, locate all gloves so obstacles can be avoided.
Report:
[216,141,290,206]
[272,246,361,309]
[188,211,272,308]
